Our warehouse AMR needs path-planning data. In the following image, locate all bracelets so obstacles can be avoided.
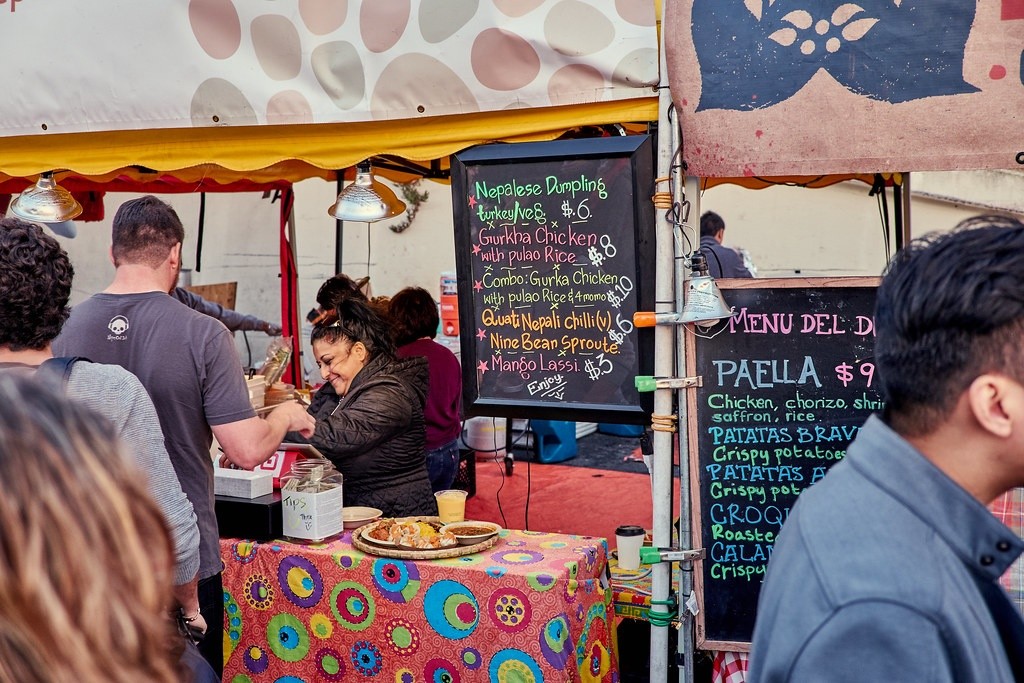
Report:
[181,607,200,621]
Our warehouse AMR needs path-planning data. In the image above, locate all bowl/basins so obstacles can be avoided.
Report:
[264,383,295,405]
[440,521,502,544]
[242,375,266,408]
[342,507,383,528]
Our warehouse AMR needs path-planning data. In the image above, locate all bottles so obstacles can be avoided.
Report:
[280,459,344,544]
[530,419,578,463]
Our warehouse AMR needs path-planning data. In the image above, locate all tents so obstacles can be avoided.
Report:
[667,0,1024,683]
[0,0,665,683]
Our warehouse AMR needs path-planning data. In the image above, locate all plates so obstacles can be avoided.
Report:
[362,522,405,547]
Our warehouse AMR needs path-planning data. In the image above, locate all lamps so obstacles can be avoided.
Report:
[676,248,740,330]
[327,158,406,223]
[10,173,83,224]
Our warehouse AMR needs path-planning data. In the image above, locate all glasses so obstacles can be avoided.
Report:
[315,315,360,342]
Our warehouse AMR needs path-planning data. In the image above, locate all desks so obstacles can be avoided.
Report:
[218,522,616,683]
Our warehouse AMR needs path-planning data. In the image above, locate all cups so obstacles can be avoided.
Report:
[615,525,646,571]
[434,490,468,521]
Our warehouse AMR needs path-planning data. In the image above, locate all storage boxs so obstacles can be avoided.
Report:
[212,467,274,500]
[450,450,476,499]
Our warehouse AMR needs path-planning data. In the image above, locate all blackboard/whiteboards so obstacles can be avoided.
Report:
[687,277,886,653]
[451,135,655,426]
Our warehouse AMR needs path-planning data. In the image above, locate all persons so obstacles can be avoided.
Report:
[699,211,752,278]
[746,213,1024,683]
[0,195,462,683]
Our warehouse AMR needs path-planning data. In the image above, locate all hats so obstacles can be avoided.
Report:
[306,273,370,327]
